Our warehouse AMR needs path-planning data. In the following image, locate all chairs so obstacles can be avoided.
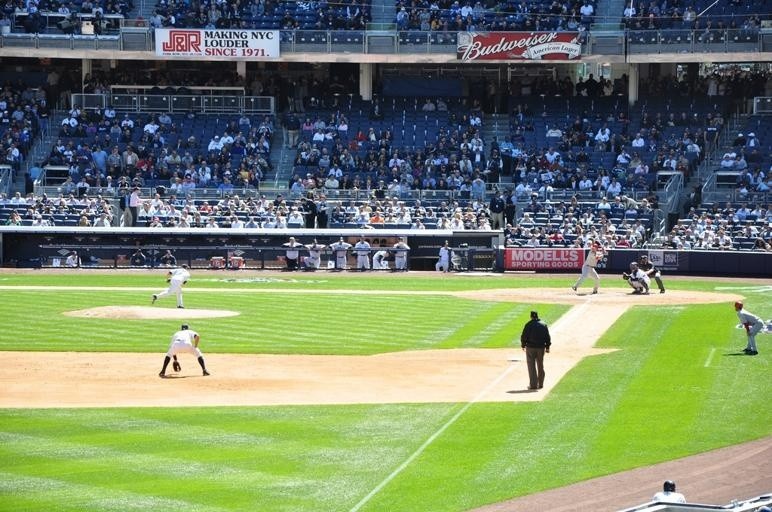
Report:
[0,0,772,257]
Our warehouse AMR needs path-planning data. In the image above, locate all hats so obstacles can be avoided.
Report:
[663,480,676,492]
[530,311,538,318]
[735,300,743,308]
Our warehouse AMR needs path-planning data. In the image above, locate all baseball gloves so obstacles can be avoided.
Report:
[623,272,630,280]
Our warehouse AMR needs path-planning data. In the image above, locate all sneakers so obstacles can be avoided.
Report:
[177,305,184,308]
[202,369,210,376]
[151,294,157,304]
[159,370,165,378]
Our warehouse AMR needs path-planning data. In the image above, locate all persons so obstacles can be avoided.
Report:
[572,243,606,294]
[638,256,665,294]
[159,324,210,376]
[622,261,650,294]
[151,263,191,308]
[652,479,686,504]
[734,301,766,355]
[1,0,771,278]
[520,309,550,390]
